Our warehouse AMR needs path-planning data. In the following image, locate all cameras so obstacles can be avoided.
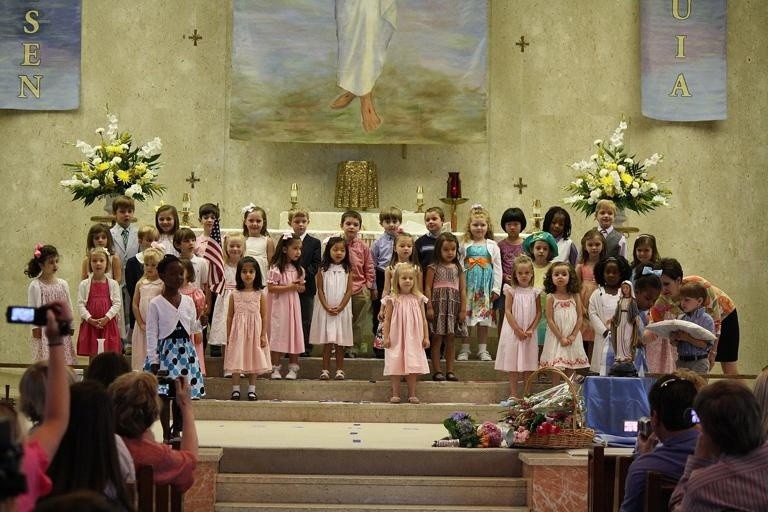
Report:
[157,370,183,398]
[683,408,700,425]
[621,416,653,436]
[6,305,47,326]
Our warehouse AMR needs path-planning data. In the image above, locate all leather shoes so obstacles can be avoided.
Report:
[446,372,457,381]
[248,392,257,400]
[231,391,240,400]
[432,372,444,380]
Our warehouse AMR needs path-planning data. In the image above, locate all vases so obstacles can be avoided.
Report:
[104,194,121,215]
[615,210,626,226]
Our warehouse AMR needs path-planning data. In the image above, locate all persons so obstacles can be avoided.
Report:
[0,300,199,512]
[177,257,206,374]
[494,254,542,405]
[619,367,767,510]
[329,3,399,128]
[266,232,305,379]
[587,255,632,376]
[223,256,272,401]
[544,262,589,387]
[77,248,122,363]
[24,245,77,365]
[610,258,739,379]
[82,198,661,361]
[309,237,353,380]
[383,262,430,403]
[424,232,466,381]
[142,254,206,442]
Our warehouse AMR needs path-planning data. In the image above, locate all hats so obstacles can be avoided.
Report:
[521,231,559,261]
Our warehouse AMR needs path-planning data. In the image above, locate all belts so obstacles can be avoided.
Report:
[680,354,709,362]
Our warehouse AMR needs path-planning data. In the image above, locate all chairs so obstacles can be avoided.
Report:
[588,446,677,512]
[125,436,184,511]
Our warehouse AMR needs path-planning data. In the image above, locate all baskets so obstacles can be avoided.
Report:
[513,366,595,449]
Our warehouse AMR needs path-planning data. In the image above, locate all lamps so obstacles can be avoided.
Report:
[526,199,541,234]
[286,183,299,211]
[334,160,379,212]
[414,186,426,213]
[178,192,197,228]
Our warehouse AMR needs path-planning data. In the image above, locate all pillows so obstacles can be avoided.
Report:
[645,319,718,341]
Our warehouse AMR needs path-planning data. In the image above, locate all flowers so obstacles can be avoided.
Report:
[58,101,168,208]
[431,375,587,449]
[558,119,676,221]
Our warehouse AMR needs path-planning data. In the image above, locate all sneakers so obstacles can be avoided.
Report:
[407,396,420,404]
[285,371,296,380]
[477,350,492,361]
[457,350,469,361]
[223,373,232,378]
[391,396,401,403]
[270,370,281,379]
[334,369,345,380]
[320,369,330,380]
